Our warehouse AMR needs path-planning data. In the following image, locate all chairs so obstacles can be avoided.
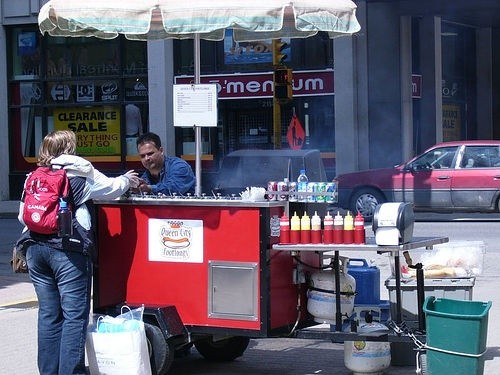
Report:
[465,159,479,167]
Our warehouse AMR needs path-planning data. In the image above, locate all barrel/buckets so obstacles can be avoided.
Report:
[347,258,379,305]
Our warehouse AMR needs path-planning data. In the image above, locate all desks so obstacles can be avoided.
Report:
[272,236,449,335]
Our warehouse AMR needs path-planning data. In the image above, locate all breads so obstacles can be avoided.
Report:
[424,264,459,279]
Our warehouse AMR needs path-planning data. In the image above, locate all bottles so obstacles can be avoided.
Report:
[297,169,309,184]
[279,211,290,244]
[57,198,73,237]
[323,210,334,244]
[344,210,354,243]
[290,211,303,244]
[310,210,321,244]
[354,211,365,244]
[301,211,310,244]
[334,211,344,244]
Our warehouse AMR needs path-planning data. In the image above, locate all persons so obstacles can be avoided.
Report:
[136,133,196,196]
[18,131,141,375]
[126,104,143,138]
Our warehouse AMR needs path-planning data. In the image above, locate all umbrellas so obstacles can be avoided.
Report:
[37,0,361,196]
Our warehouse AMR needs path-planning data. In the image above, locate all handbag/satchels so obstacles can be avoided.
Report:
[11,246,29,274]
[86,305,151,375]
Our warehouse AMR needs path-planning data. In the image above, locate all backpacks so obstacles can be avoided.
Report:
[23,164,72,235]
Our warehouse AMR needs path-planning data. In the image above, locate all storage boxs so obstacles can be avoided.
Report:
[385,273,476,366]
[331,299,390,344]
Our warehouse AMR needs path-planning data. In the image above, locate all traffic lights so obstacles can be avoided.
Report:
[273,38,288,66]
[273,68,292,99]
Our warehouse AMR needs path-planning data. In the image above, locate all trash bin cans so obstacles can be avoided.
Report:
[423,296,492,375]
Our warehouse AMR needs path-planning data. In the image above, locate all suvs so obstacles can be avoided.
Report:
[331,139,500,223]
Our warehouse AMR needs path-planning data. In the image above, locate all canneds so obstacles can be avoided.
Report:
[268,181,297,202]
[307,181,338,204]
